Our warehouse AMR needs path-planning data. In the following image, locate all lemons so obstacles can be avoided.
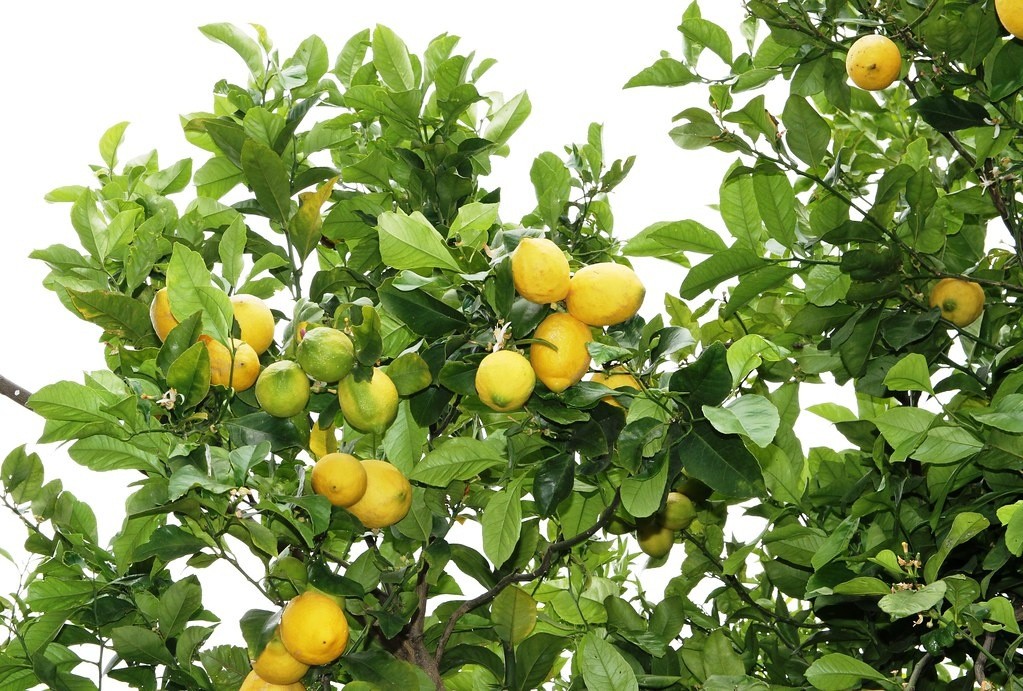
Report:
[846,34,902,91]
[150,239,1023,691]
[995,0,1023,41]
